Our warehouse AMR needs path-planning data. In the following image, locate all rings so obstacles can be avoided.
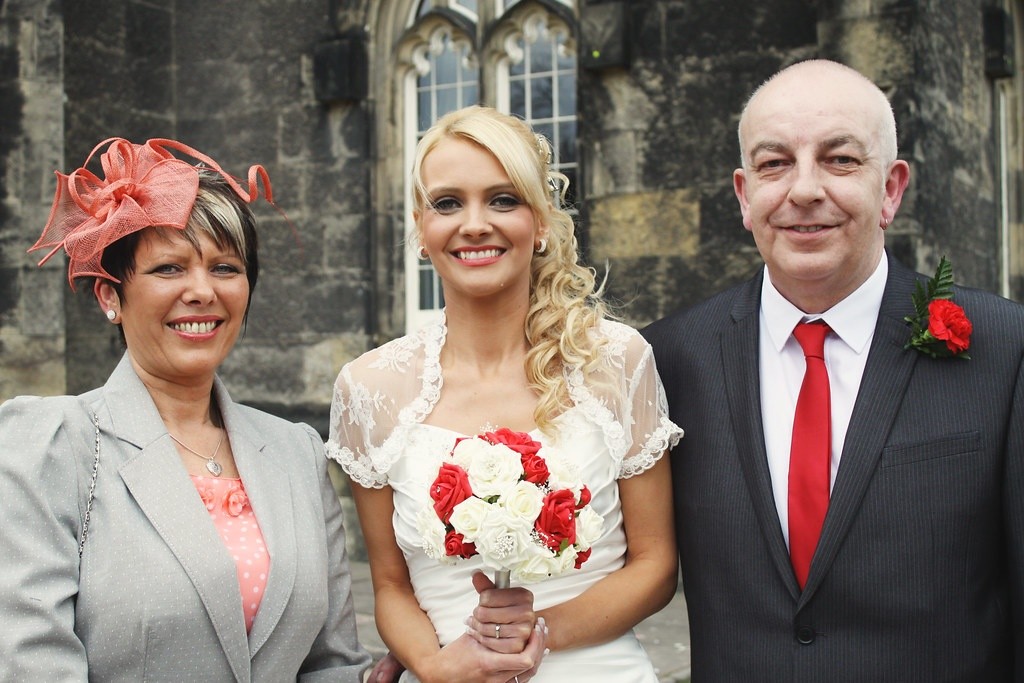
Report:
[514,676,519,683]
[496,623,501,639]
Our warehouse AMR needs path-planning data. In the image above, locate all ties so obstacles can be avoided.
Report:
[788,322,831,589]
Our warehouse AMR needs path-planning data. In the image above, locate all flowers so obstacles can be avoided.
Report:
[900,253,974,365]
[414,427,605,588]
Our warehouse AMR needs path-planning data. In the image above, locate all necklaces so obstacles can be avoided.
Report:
[168,406,223,477]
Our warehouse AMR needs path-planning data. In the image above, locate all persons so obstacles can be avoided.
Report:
[637,58,1023,683]
[0,139,373,683]
[325,105,685,683]
